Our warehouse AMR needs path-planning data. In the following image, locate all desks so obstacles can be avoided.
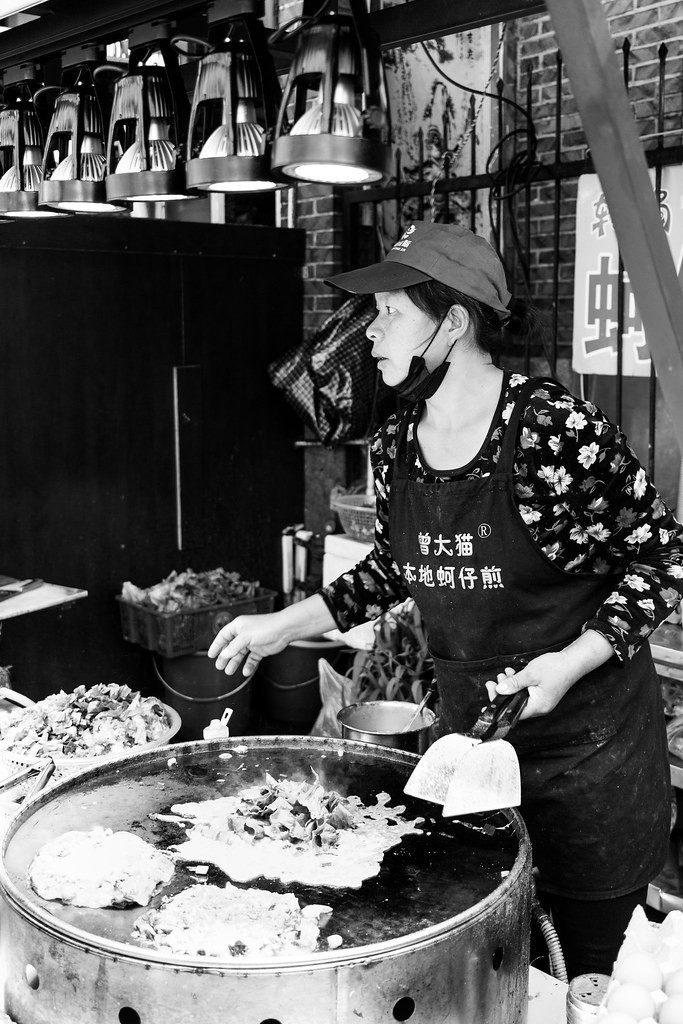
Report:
[0,712,606,1023]
[1,568,91,643]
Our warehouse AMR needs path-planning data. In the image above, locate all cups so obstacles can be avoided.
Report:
[337,700,438,757]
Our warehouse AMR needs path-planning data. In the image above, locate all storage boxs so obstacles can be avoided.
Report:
[117,586,279,658]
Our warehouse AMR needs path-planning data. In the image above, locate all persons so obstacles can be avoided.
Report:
[207,223,682,980]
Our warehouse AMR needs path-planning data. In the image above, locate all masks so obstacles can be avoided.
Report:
[390,305,458,403]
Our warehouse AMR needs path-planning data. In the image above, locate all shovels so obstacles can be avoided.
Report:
[403,657,529,806]
[441,686,530,819]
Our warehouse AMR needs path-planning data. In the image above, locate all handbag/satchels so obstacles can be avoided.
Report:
[265,288,389,451]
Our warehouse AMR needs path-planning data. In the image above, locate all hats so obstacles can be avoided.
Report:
[322,219,512,328]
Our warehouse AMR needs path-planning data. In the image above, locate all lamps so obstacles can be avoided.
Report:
[271,0,390,188]
[37,70,134,215]
[184,24,298,197]
[105,47,206,204]
[0,90,76,218]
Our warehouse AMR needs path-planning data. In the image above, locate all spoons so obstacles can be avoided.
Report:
[400,677,437,734]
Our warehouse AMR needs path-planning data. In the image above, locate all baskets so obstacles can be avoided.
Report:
[115,579,271,659]
[331,495,376,544]
